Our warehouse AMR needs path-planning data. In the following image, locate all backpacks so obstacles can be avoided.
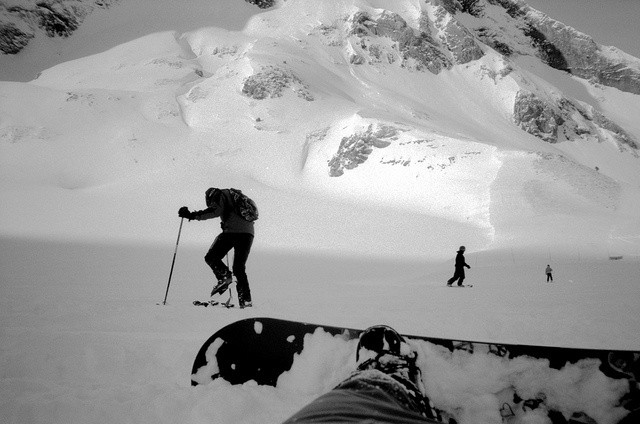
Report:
[229,188,258,222]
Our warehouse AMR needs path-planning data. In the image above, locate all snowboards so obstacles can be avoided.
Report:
[190,318,640,424]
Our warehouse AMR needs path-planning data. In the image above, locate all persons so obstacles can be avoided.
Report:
[544,263,553,283]
[448,246,471,290]
[179,186,259,306]
[276,326,434,423]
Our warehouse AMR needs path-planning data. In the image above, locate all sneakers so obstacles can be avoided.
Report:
[212,274,232,296]
[355,324,444,424]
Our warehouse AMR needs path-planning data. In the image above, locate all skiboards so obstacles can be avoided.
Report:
[192,300,253,309]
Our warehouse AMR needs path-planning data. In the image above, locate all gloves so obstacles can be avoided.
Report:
[178,205,194,222]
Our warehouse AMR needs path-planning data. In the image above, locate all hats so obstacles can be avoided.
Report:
[204,187,219,207]
